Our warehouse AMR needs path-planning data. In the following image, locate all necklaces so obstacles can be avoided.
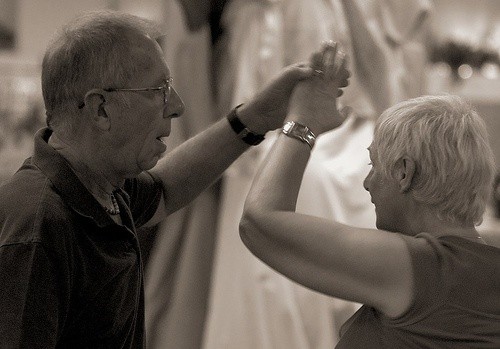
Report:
[102,192,120,215]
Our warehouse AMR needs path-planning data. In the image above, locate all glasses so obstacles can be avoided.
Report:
[77,77,173,111]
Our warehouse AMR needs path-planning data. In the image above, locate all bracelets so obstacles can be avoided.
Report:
[227,102,265,145]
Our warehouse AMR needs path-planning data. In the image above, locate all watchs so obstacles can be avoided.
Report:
[279,120,316,149]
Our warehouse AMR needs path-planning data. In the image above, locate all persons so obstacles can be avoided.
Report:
[239,40,500,349]
[0,10,324,349]
